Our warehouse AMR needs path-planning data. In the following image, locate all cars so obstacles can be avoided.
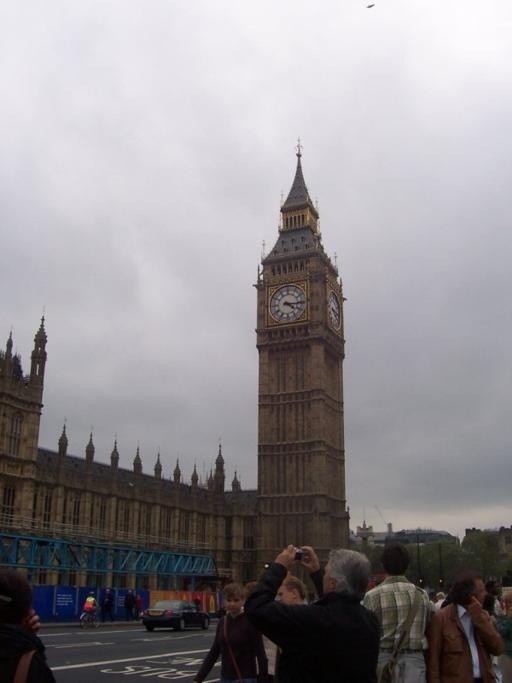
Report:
[139,600,211,631]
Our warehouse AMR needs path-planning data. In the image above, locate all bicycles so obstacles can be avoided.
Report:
[80,605,101,628]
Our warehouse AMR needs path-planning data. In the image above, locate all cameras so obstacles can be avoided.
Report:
[294,551,303,559]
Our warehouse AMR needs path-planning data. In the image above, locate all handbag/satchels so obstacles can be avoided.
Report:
[376,662,394,683]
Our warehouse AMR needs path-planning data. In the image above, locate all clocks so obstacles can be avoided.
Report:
[328,291,340,330]
[269,284,307,322]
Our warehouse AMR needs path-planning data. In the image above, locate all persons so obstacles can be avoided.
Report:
[0,564,59,682]
[192,540,512,683]
[78,584,148,630]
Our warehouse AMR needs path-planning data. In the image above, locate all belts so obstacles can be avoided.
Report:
[379,648,421,653]
